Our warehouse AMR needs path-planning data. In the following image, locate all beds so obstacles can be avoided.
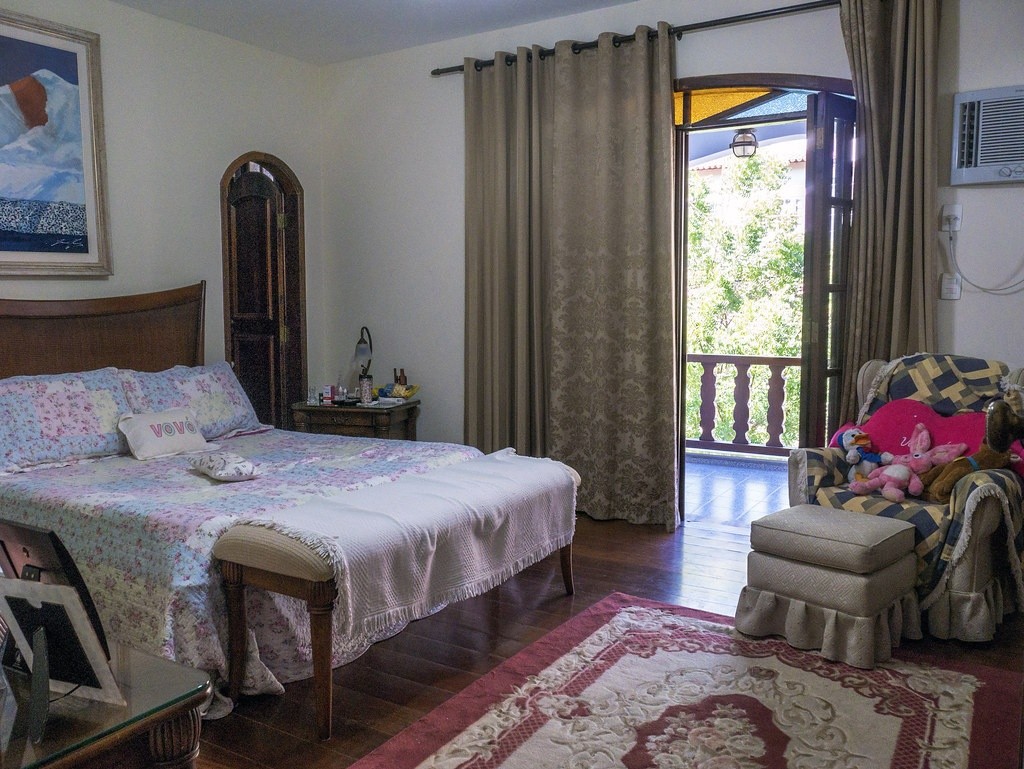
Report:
[0,279,487,720]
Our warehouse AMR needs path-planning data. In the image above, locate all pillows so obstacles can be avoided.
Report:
[116,360,276,442]
[118,406,222,462]
[0,367,134,476]
[186,452,264,483]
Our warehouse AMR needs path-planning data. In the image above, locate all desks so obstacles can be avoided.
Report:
[1,635,216,769]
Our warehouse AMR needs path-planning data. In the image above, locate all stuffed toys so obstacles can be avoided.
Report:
[837,427,894,482]
[849,422,968,504]
[907,437,1023,504]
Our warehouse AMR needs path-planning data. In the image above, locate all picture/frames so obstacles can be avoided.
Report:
[0,8,116,280]
[0,518,114,661]
[0,575,129,749]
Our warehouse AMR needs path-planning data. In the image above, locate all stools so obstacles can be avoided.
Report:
[734,504,926,671]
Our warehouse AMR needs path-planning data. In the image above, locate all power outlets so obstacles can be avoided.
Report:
[941,203,963,232]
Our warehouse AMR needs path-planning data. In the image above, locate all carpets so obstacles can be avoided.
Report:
[345,590,1024,768]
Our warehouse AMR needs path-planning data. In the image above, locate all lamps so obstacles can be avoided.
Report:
[353,326,373,377]
[728,129,760,160]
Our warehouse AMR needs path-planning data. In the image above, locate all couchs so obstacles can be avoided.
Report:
[787,351,1024,646]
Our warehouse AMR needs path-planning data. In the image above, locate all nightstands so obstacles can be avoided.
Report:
[290,396,424,441]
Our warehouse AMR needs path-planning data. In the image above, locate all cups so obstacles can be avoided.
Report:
[359,374,374,403]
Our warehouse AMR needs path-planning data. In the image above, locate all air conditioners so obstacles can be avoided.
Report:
[949,82,1024,187]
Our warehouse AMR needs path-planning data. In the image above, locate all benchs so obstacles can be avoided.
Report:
[214,445,583,743]
